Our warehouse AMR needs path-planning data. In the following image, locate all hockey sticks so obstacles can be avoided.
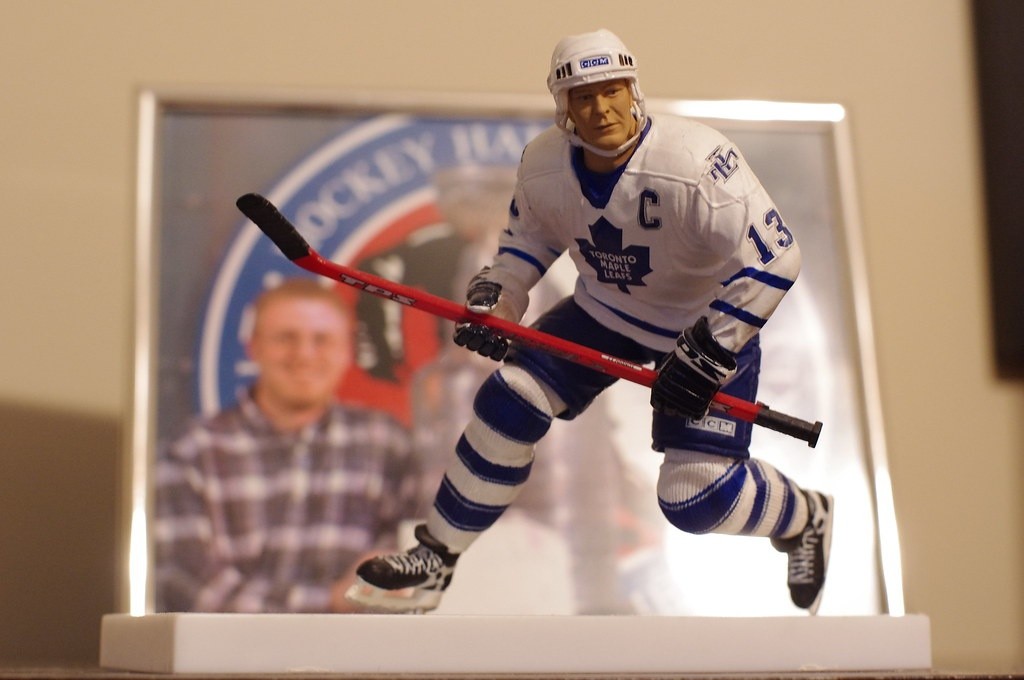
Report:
[233,188,826,453]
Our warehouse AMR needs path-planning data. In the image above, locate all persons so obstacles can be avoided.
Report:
[344,28,835,616]
[151,278,426,614]
